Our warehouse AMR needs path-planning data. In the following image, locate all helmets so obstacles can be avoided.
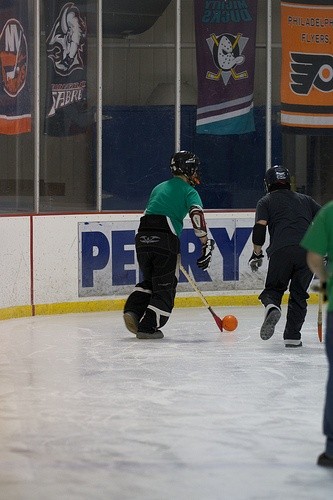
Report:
[170,151,200,176]
[265,165,290,191]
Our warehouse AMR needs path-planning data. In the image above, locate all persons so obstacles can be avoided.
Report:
[299,201,333,467]
[123,151,215,339]
[248,165,325,346]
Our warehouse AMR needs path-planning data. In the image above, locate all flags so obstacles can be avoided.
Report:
[279,0,333,136]
[194,0,257,135]
[41,0,90,136]
[0,0,32,134]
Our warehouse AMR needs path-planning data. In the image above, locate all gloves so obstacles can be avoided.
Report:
[249,248,264,272]
[196,239,214,271]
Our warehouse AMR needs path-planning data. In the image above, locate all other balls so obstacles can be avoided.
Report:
[221,315,238,331]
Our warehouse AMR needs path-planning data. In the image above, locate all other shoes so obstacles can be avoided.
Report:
[124,312,139,335]
[285,341,303,348]
[260,310,282,340]
[318,452,333,468]
[136,329,165,340]
